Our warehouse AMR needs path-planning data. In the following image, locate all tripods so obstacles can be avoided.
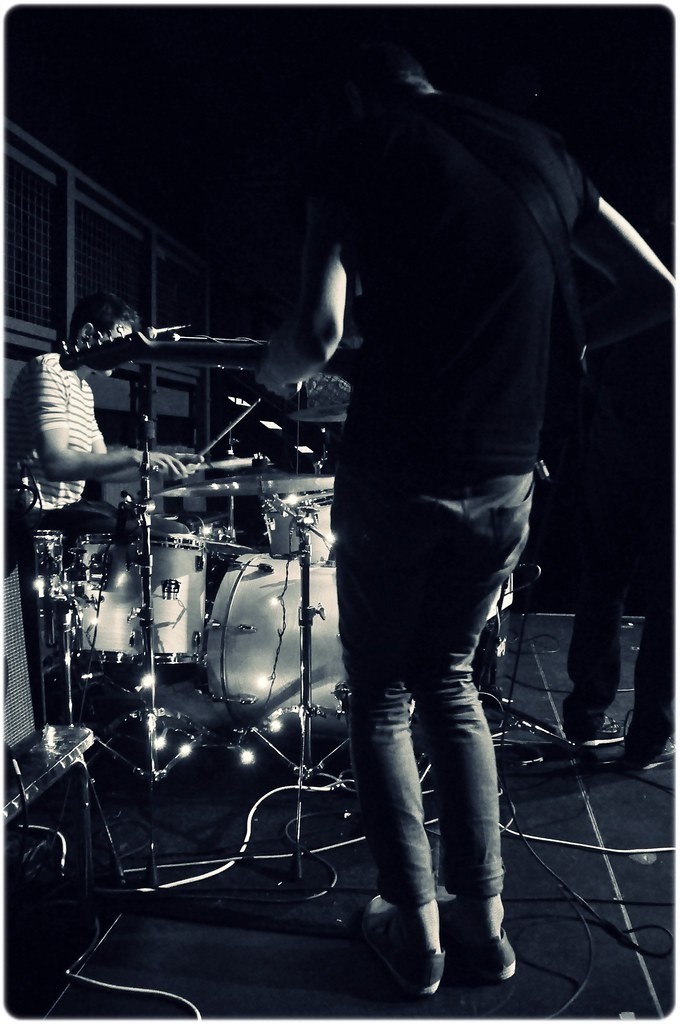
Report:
[86,442,333,897]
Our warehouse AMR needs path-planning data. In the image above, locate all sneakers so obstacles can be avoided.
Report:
[620,737,675,771]
[565,715,628,746]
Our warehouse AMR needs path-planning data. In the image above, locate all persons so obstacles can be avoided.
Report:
[255,10,673,995]
[6,290,204,542]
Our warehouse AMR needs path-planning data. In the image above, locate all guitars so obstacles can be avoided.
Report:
[56,318,334,382]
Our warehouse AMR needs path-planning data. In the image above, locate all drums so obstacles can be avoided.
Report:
[72,533,209,666]
[207,552,417,743]
[261,488,338,564]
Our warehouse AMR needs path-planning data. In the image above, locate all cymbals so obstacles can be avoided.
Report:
[150,456,335,499]
[200,455,275,471]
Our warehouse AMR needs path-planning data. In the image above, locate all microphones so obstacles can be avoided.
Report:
[145,324,194,340]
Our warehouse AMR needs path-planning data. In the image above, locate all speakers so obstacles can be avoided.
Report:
[5,537,46,769]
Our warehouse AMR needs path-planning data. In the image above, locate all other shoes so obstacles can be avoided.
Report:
[434,900,516,981]
[360,894,446,997]
